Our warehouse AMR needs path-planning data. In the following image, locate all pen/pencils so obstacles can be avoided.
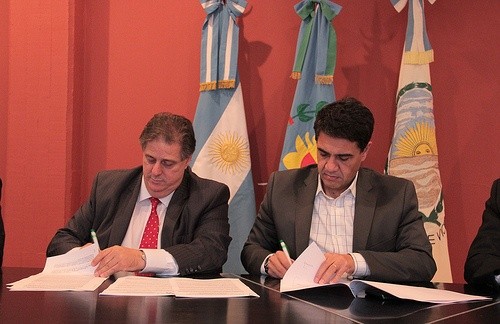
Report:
[91,229,100,249]
[279,239,292,264]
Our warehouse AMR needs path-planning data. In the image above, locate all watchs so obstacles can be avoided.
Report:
[142,253,146,260]
[264,257,270,274]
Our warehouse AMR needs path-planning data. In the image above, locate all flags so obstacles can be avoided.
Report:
[189,0,257,274]
[387,0,452,282]
[279,0,343,172]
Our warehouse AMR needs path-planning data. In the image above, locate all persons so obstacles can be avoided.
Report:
[47,112,232,277]
[241,98,438,281]
[464,178,500,287]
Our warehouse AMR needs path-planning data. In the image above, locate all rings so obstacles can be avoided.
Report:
[332,263,338,269]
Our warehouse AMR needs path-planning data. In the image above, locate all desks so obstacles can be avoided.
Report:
[0,254,500,324]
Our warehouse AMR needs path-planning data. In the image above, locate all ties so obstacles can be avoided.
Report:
[135,197,162,277]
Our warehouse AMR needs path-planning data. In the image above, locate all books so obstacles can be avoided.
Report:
[280,242,491,304]
